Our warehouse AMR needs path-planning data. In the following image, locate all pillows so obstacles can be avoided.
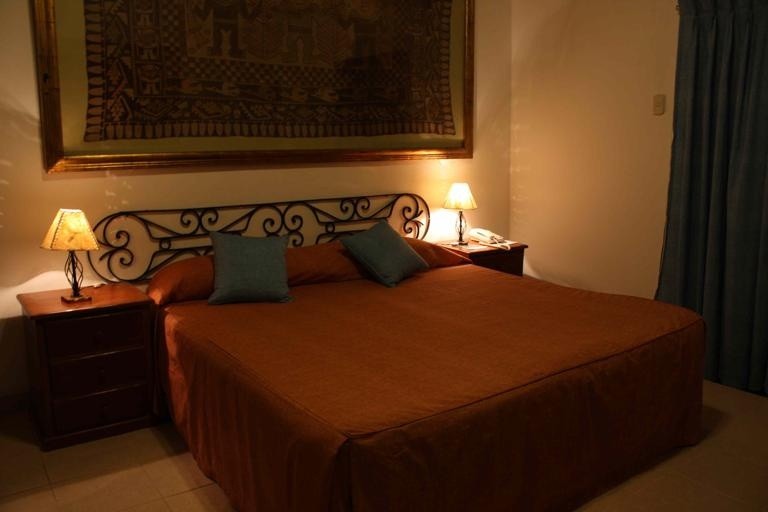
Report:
[207,230,291,305]
[338,219,432,287]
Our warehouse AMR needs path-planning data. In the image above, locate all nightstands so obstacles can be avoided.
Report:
[14,281,155,452]
[431,233,529,277]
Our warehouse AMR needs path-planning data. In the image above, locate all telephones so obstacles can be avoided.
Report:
[470,228,504,244]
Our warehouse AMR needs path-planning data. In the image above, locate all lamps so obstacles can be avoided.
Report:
[441,182,479,246]
[39,207,102,304]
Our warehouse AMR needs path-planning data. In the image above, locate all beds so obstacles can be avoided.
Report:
[86,192,706,512]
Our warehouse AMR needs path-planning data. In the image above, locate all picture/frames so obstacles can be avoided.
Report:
[26,0,475,181]
